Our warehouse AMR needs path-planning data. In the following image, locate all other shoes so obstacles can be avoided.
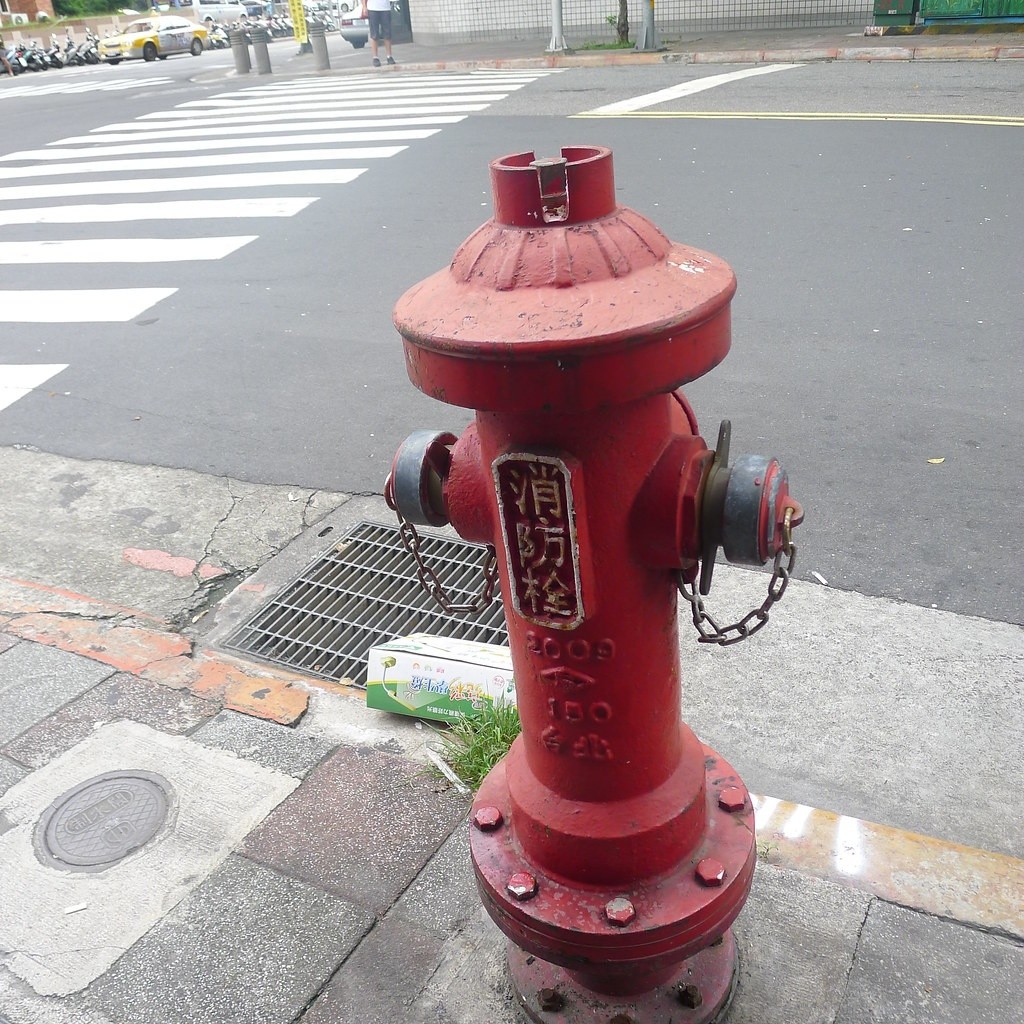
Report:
[372,58,382,67]
[387,57,396,64]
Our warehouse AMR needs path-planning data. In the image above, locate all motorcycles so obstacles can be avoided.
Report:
[212,6,336,49]
[0,28,101,76]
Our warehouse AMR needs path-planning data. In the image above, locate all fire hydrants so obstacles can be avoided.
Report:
[385,146,805,1024]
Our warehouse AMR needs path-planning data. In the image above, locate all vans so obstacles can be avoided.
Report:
[168,0,248,24]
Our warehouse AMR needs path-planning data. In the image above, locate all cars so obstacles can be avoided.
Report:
[240,0,362,16]
[339,5,383,49]
[97,16,211,65]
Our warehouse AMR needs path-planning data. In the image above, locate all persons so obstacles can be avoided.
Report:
[361,0,396,67]
